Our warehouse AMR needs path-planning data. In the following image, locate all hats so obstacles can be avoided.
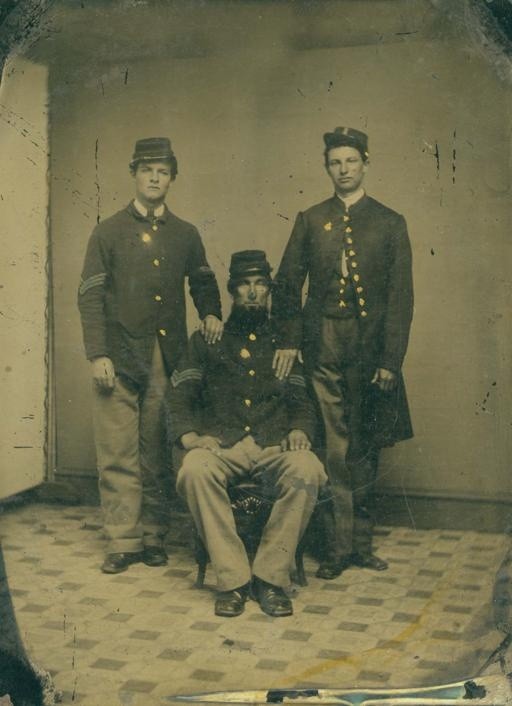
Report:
[323,126,369,152]
[229,251,272,279]
[129,137,173,169]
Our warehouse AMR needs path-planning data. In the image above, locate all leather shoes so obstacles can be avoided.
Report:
[102,551,133,573]
[251,575,292,616]
[316,558,351,579]
[351,552,388,571]
[144,546,168,566]
[215,581,251,616]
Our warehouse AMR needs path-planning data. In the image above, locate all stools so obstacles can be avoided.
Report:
[188,483,313,593]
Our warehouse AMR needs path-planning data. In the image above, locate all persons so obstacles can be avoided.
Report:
[271,127,414,578]
[78,137,225,572]
[163,249,329,616]
